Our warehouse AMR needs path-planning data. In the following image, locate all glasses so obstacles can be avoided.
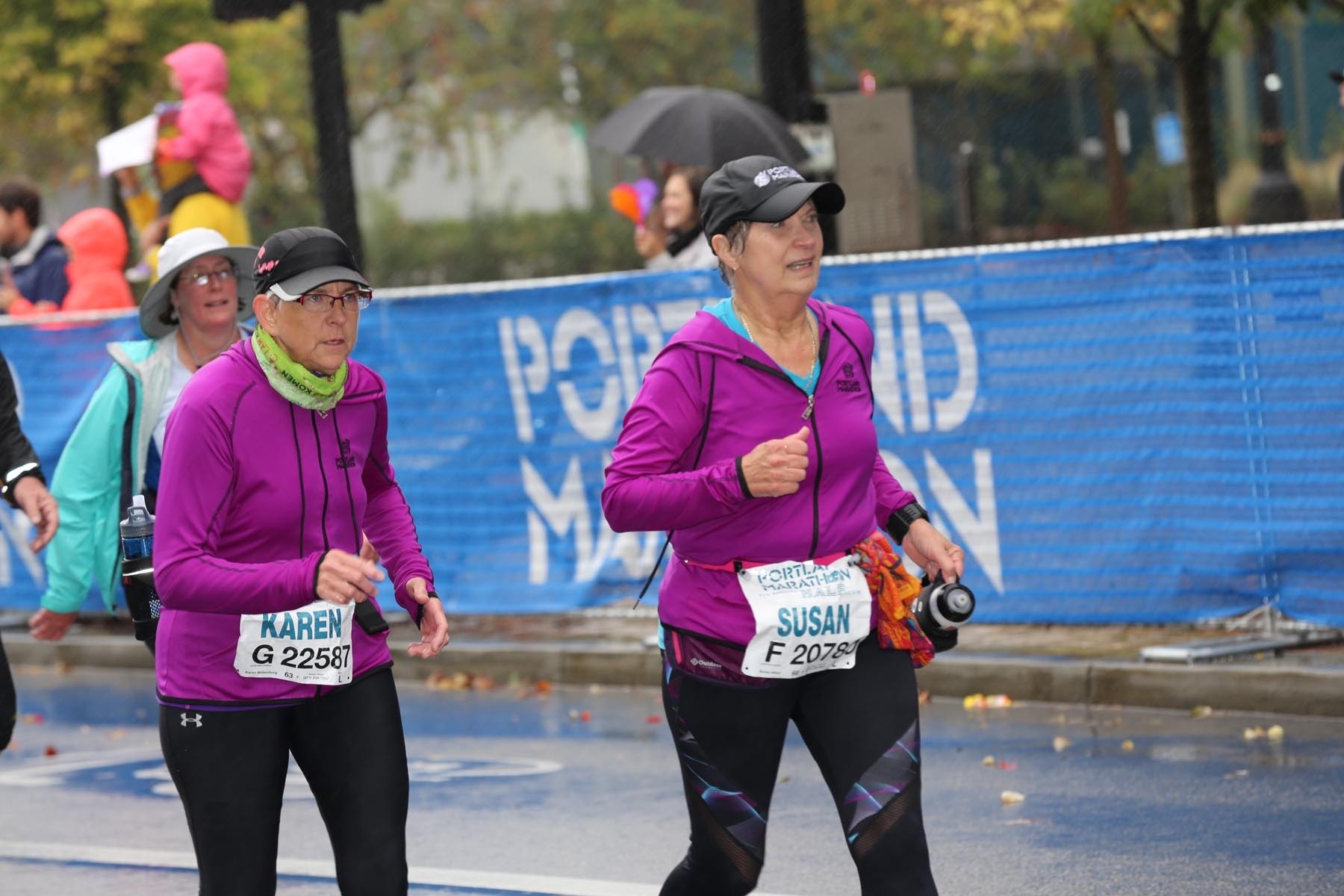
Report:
[175,266,238,286]
[301,289,372,310]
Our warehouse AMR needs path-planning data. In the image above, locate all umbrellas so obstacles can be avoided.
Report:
[594,85,808,174]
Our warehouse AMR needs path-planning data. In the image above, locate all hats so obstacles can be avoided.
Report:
[136,228,260,340]
[698,156,847,249]
[250,227,371,302]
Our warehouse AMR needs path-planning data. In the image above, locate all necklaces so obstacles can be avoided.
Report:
[736,296,816,390]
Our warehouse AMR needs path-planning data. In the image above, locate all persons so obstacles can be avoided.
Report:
[0,44,450,896]
[603,163,965,896]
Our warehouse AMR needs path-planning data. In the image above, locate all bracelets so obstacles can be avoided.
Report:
[888,504,928,547]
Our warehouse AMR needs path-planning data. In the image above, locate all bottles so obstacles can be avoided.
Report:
[119,494,161,617]
[910,567,977,639]
